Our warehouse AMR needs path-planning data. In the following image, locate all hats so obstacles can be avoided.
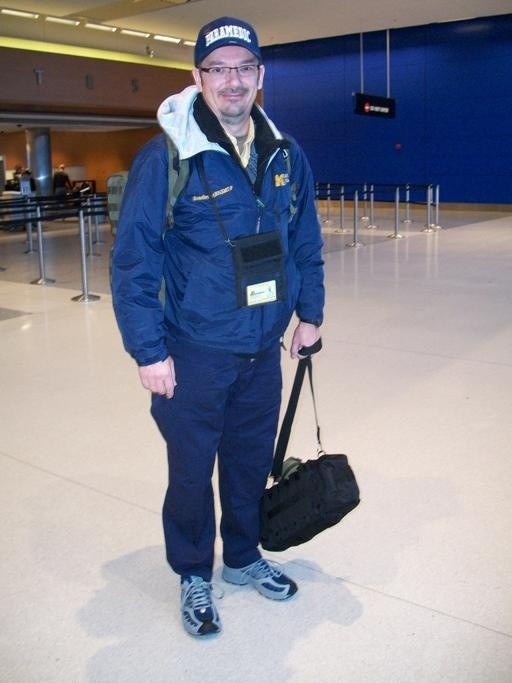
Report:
[194,17,263,67]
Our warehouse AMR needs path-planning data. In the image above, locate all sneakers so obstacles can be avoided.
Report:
[181,575,221,637]
[222,558,297,600]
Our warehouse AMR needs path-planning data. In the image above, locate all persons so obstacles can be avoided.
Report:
[13,165,24,181]
[52,163,72,193]
[107,15,328,636]
[17,167,36,190]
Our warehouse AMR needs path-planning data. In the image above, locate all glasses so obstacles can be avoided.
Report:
[200,65,257,77]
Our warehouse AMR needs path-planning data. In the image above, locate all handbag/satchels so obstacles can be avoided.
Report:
[231,231,285,309]
[259,454,358,551]
